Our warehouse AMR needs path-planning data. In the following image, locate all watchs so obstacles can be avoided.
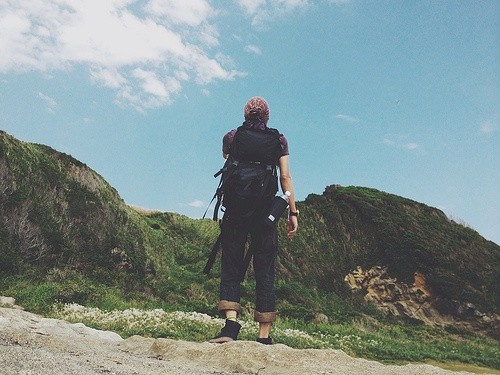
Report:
[287,209,300,217]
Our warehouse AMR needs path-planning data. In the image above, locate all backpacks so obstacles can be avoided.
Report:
[214,126,288,231]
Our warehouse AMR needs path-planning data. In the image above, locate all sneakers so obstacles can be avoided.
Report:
[256,336,272,345]
[209,319,242,343]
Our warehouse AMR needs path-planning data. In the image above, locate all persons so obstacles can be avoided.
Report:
[199,98,301,344]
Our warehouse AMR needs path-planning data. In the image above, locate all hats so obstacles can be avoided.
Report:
[244,96,270,121]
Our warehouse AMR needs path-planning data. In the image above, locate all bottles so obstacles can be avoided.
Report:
[280,191,291,203]
